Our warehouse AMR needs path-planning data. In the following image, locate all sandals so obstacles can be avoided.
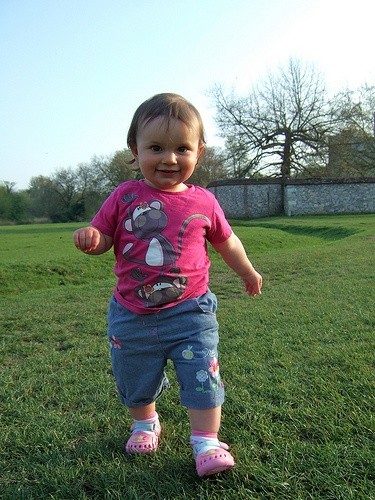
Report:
[192,440,236,478]
[125,421,163,454]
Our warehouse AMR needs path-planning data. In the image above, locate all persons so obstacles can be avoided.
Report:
[72,93,262,478]
